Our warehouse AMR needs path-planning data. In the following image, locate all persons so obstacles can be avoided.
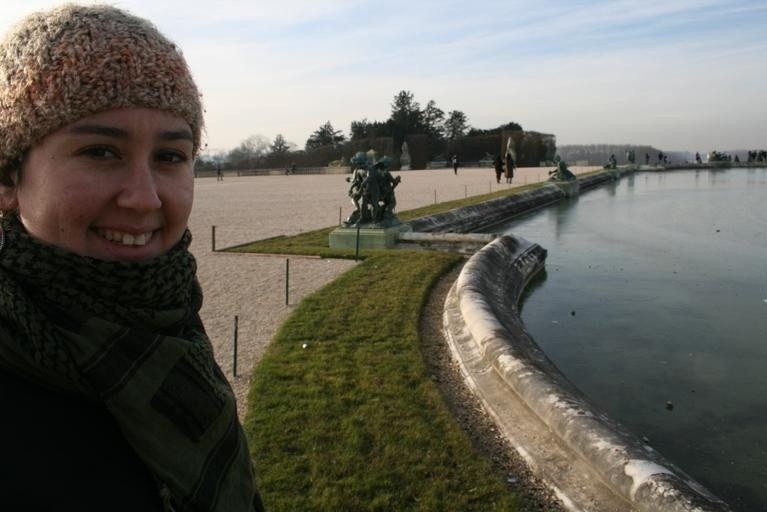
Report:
[503,152,516,184]
[750,149,756,162]
[450,154,458,175]
[694,151,699,162]
[216,165,224,182]
[746,149,752,162]
[0,2,265,511]
[491,155,505,184]
[343,153,401,227]
[733,154,738,162]
[662,154,667,165]
[657,151,664,165]
[644,153,649,165]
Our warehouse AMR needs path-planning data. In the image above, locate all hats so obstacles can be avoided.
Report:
[1,4,203,172]
